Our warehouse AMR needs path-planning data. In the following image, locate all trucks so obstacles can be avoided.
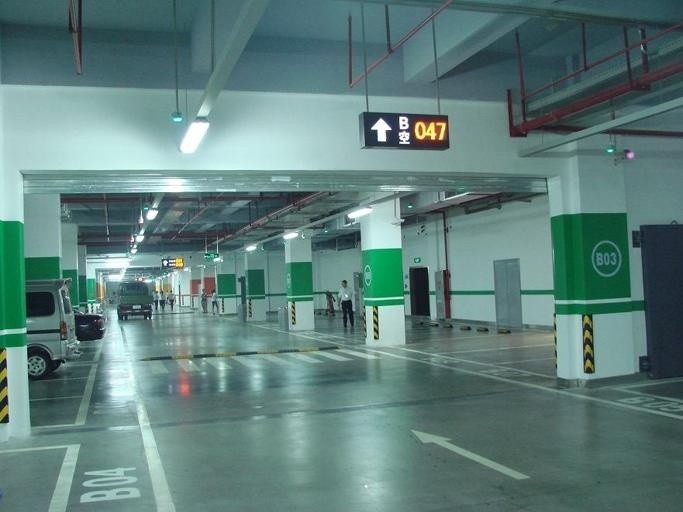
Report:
[117,281,154,321]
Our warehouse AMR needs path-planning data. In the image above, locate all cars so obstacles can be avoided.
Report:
[73,308,106,342]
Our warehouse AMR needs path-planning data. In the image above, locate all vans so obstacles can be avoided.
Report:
[26,279,84,381]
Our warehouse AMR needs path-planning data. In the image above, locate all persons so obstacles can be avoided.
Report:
[158,291,165,310]
[200,288,208,313]
[165,290,176,312]
[336,279,354,330]
[211,289,220,315]
[151,290,155,302]
[153,291,159,310]
